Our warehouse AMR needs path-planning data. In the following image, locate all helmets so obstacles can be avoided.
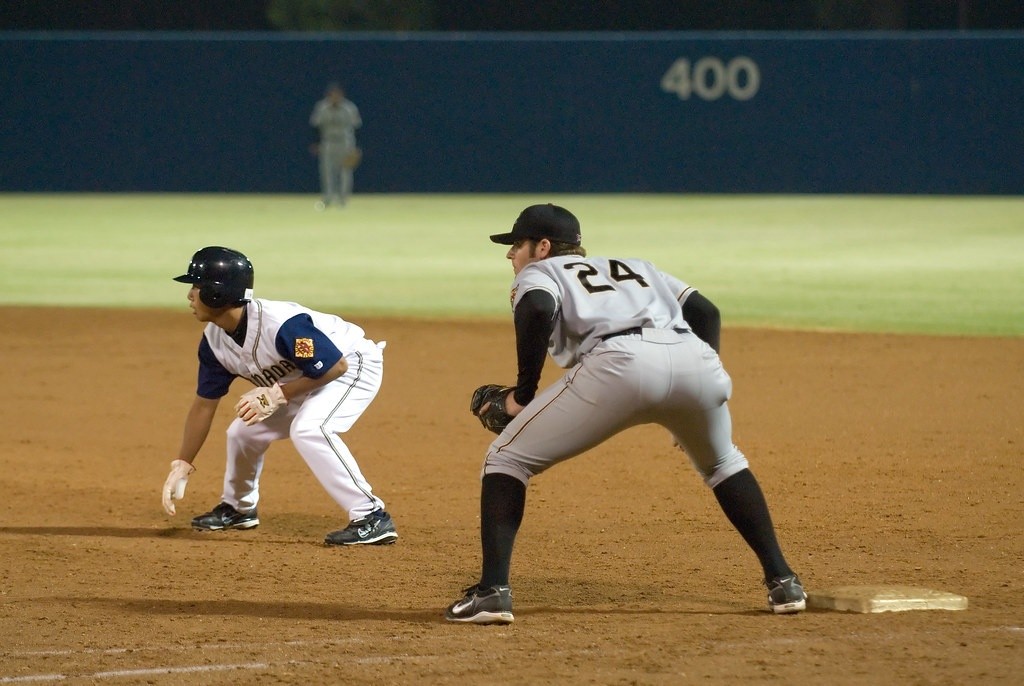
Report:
[173,246,254,308]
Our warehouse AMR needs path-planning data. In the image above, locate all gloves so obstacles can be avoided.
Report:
[235,382,288,427]
[162,459,197,515]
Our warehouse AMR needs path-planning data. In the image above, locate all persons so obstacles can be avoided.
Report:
[445,203,808,624]
[162,246,398,547]
[309,84,363,209]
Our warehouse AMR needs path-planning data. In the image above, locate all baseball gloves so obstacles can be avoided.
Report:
[471,383,517,435]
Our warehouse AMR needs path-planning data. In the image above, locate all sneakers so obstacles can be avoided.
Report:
[446,584,515,624]
[764,574,807,613]
[191,502,260,530]
[325,513,398,545]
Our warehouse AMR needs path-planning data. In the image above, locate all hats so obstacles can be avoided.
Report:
[490,203,582,245]
